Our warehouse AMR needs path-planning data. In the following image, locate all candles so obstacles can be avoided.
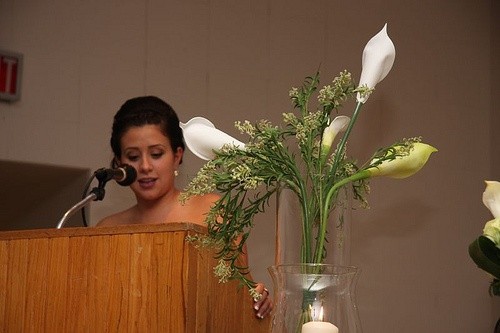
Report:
[302,306,338,333]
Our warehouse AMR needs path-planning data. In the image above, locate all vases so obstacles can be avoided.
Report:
[267,174,364,333]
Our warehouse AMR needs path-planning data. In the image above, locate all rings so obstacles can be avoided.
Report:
[263,287,270,296]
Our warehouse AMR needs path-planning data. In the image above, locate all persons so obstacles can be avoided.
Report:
[93,96,275,320]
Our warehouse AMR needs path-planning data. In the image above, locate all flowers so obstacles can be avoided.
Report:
[470,180,500,333]
[178,22,439,333]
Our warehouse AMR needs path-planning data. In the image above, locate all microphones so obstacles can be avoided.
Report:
[96,164,137,189]
